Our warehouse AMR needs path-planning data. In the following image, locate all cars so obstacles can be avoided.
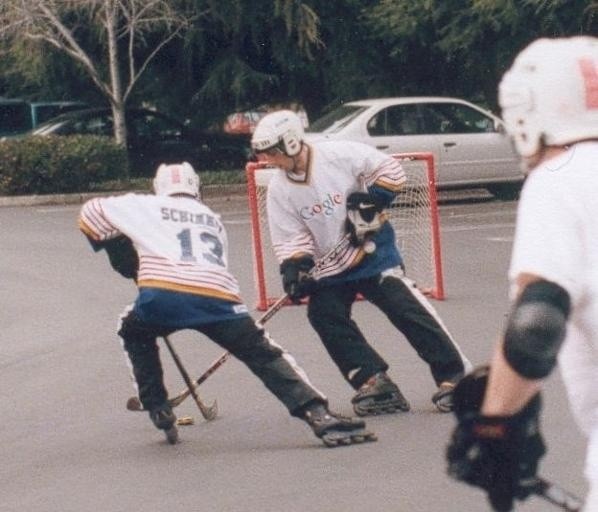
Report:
[19,105,222,185]
[260,90,541,209]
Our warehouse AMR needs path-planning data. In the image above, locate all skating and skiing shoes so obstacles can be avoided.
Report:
[352,372,410,416]
[433,381,457,412]
[304,406,376,447]
[150,403,179,444]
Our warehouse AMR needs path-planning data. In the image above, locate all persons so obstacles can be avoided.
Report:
[78,162,368,439]
[248,110,476,404]
[441,37,597,512]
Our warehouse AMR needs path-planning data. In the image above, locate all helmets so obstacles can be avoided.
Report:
[251,110,305,157]
[154,162,201,196]
[498,35,598,157]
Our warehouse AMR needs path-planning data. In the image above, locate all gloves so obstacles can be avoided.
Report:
[107,237,139,278]
[279,256,317,304]
[345,193,381,246]
[448,366,549,512]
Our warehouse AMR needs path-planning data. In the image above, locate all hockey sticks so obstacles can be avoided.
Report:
[126,231,351,411]
[134,275,217,421]
[464,439,585,512]
